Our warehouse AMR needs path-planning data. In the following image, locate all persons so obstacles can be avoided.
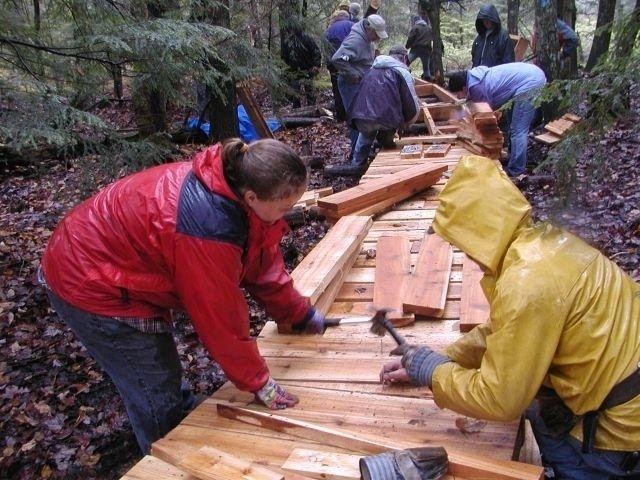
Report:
[532,1,578,72]
[42,137,326,458]
[377,154,640,480]
[470,4,514,70]
[448,60,547,178]
[324,1,435,169]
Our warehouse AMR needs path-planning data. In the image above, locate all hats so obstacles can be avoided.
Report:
[368,14,389,39]
[349,3,360,14]
[389,45,410,67]
[412,15,420,22]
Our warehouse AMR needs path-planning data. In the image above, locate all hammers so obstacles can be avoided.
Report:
[371,308,407,346]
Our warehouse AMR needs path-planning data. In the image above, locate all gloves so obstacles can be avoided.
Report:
[291,307,327,335]
[250,376,300,410]
[359,447,449,480]
[388,344,456,392]
